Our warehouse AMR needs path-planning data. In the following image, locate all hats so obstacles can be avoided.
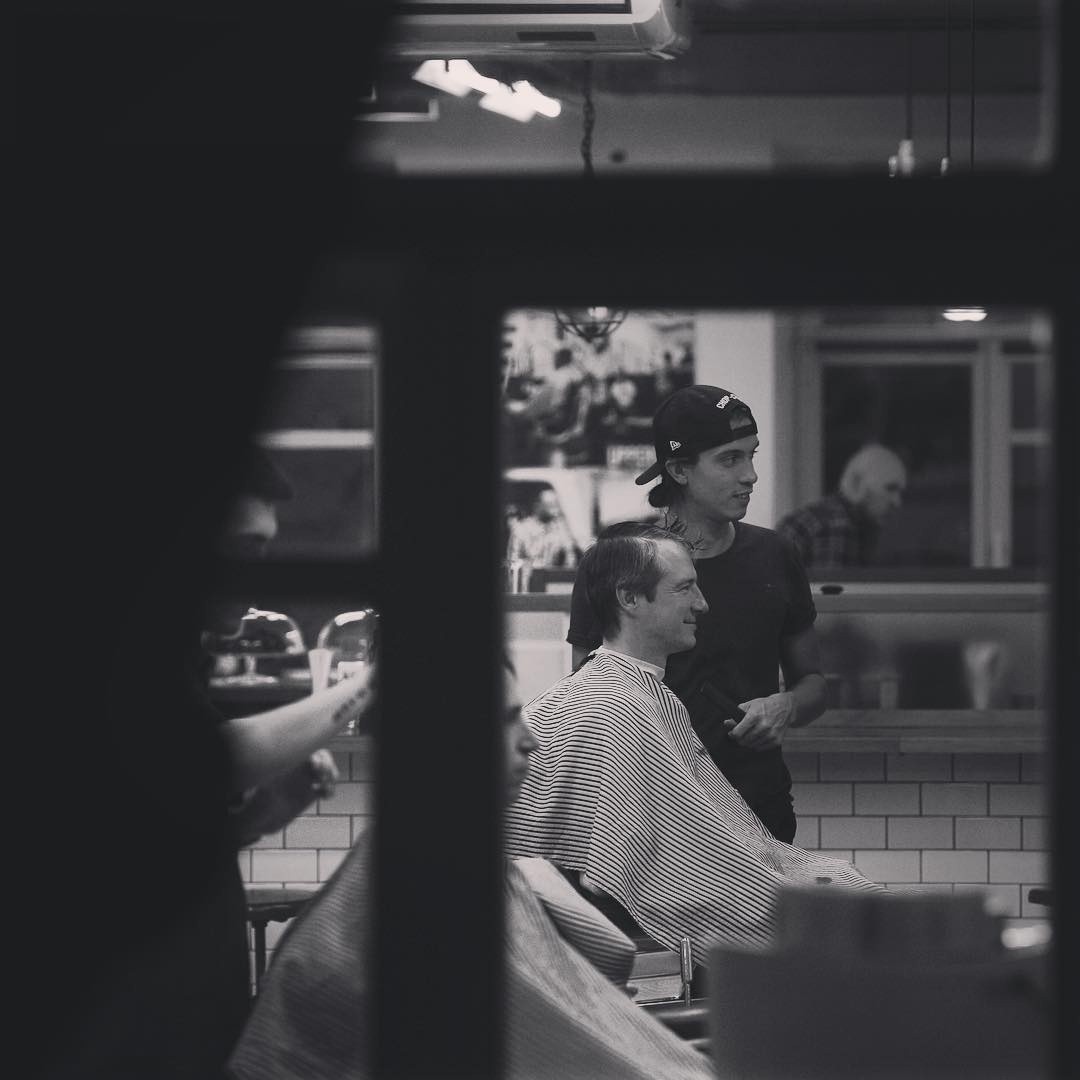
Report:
[634,383,757,486]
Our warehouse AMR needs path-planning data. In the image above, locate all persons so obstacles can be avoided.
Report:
[566,388,831,847]
[503,521,894,1000]
[774,443,913,587]
[219,448,375,847]
[224,655,714,1080]
